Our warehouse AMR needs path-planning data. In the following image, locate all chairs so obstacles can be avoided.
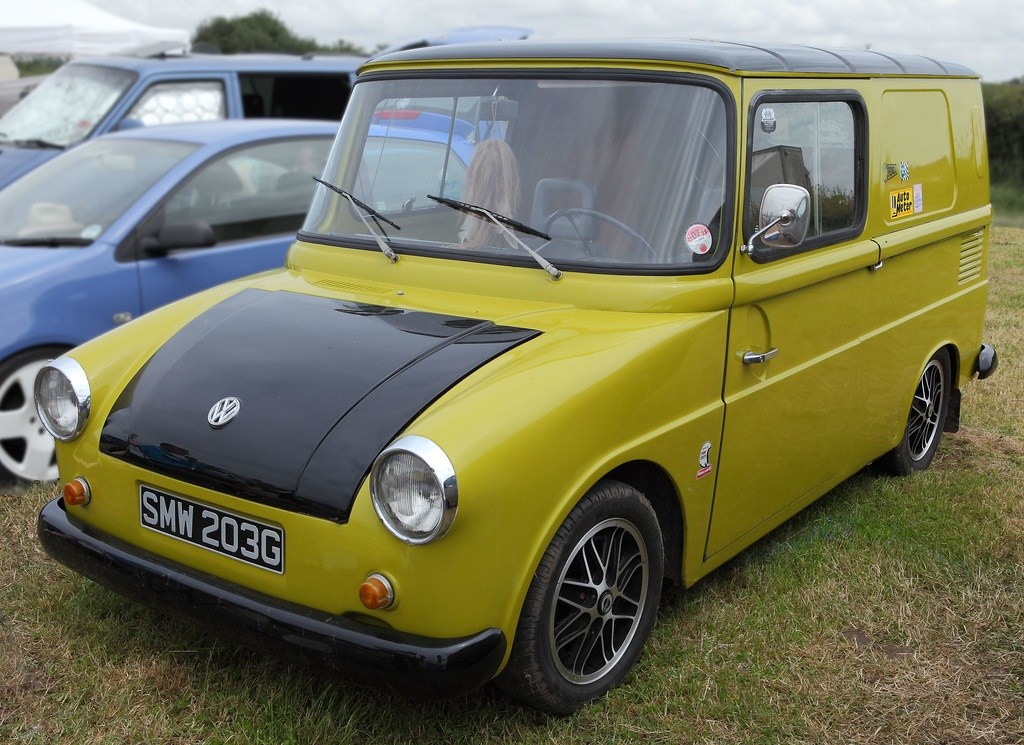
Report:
[680,185,766,259]
[517,177,597,257]
[186,164,315,223]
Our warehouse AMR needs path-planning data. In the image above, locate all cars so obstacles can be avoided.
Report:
[0,27,540,487]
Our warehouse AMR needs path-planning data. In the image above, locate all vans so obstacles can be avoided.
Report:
[35,27,995,721]
[0,48,368,183]
